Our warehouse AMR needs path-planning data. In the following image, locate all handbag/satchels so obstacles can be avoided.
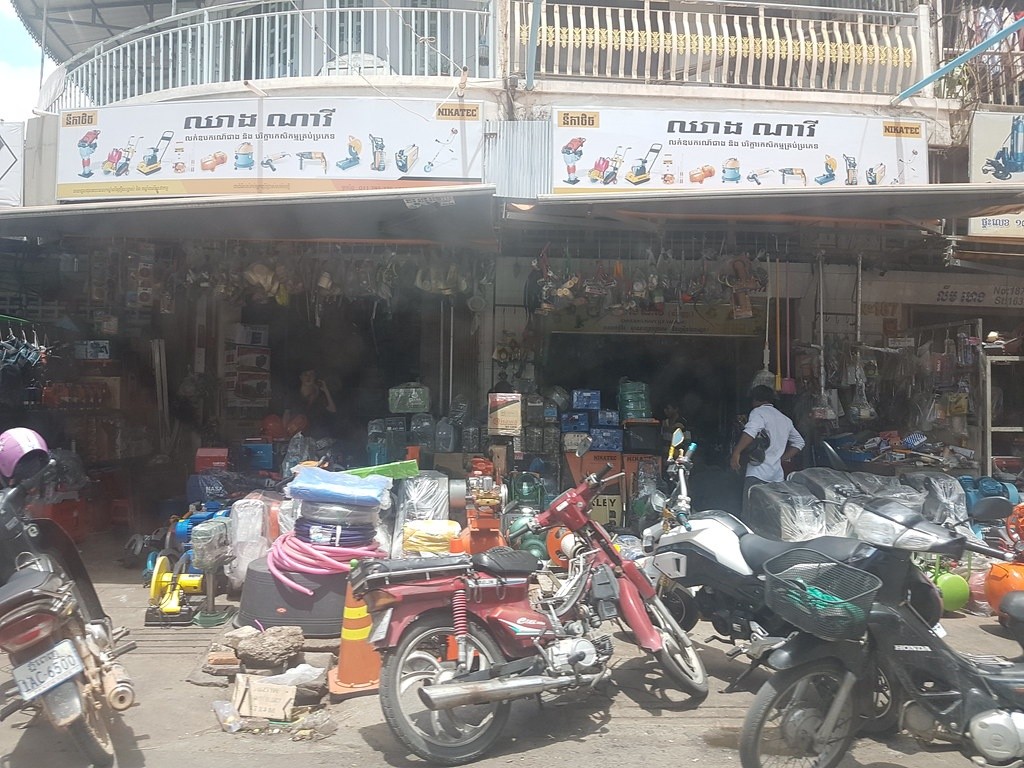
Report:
[741,431,769,466]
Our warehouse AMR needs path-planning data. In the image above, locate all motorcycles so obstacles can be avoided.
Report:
[737,438,1023,768]
[344,437,712,766]
[1,459,139,768]
[617,427,942,732]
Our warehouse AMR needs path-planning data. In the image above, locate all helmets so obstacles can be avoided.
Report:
[0,427,50,477]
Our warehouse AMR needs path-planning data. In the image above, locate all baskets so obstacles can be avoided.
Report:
[762,548,883,641]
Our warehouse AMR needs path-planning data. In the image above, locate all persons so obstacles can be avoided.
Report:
[282,362,336,441]
[729,385,805,521]
[658,397,698,514]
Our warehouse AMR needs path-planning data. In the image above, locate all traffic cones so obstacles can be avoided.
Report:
[327,581,384,698]
[434,634,480,661]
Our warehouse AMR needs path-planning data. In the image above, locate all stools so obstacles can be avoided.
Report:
[109,500,141,527]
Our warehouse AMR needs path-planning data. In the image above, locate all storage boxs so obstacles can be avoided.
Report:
[370,380,662,534]
[79,375,130,409]
[224,323,272,407]
[26,487,86,542]
[73,340,110,360]
[194,448,229,470]
[242,445,275,468]
